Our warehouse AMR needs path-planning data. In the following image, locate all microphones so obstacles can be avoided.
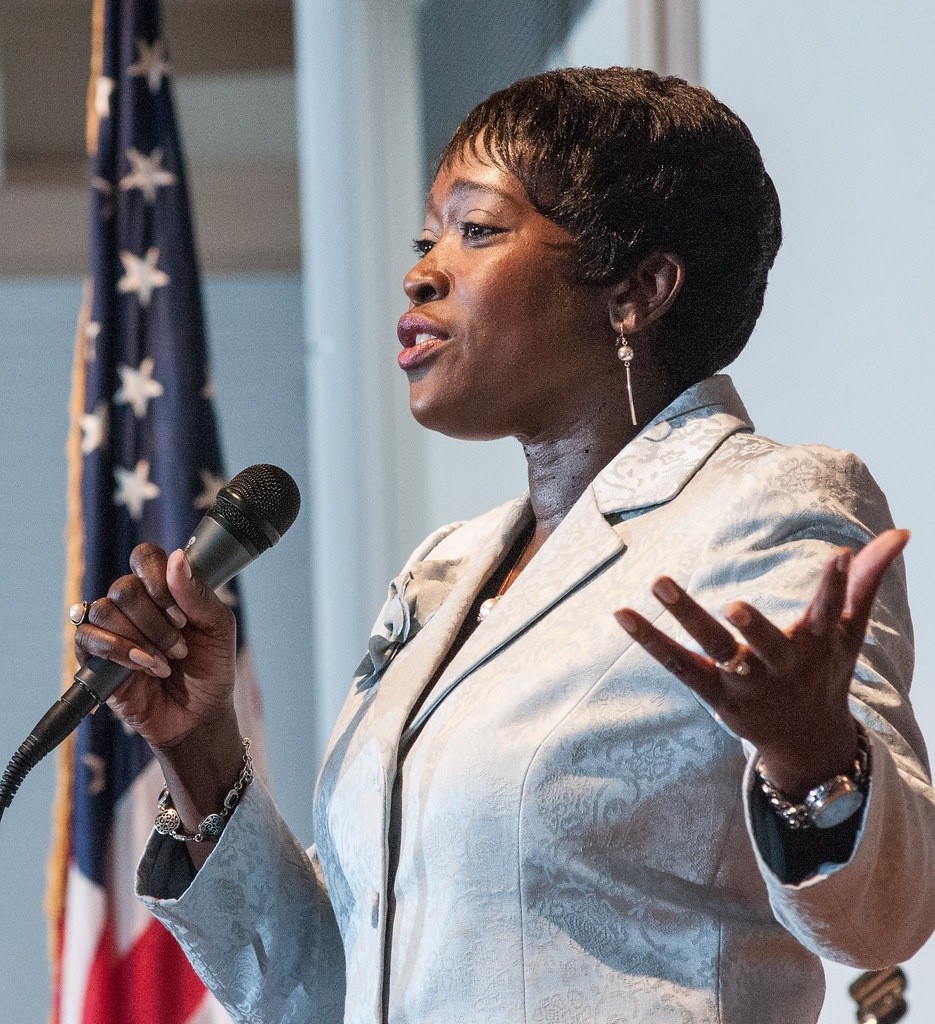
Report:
[61,463,301,718]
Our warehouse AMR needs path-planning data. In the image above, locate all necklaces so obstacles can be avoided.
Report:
[477,524,536,623]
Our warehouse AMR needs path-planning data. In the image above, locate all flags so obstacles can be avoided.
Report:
[40,0,271,1024]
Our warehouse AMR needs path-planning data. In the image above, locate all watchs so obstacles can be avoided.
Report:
[758,750,868,832]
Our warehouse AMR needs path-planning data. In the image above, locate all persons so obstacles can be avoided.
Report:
[72,66,935,1024]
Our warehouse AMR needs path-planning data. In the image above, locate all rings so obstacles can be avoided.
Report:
[715,645,750,676]
[68,600,89,626]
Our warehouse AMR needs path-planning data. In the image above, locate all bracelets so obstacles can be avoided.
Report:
[153,737,253,842]
